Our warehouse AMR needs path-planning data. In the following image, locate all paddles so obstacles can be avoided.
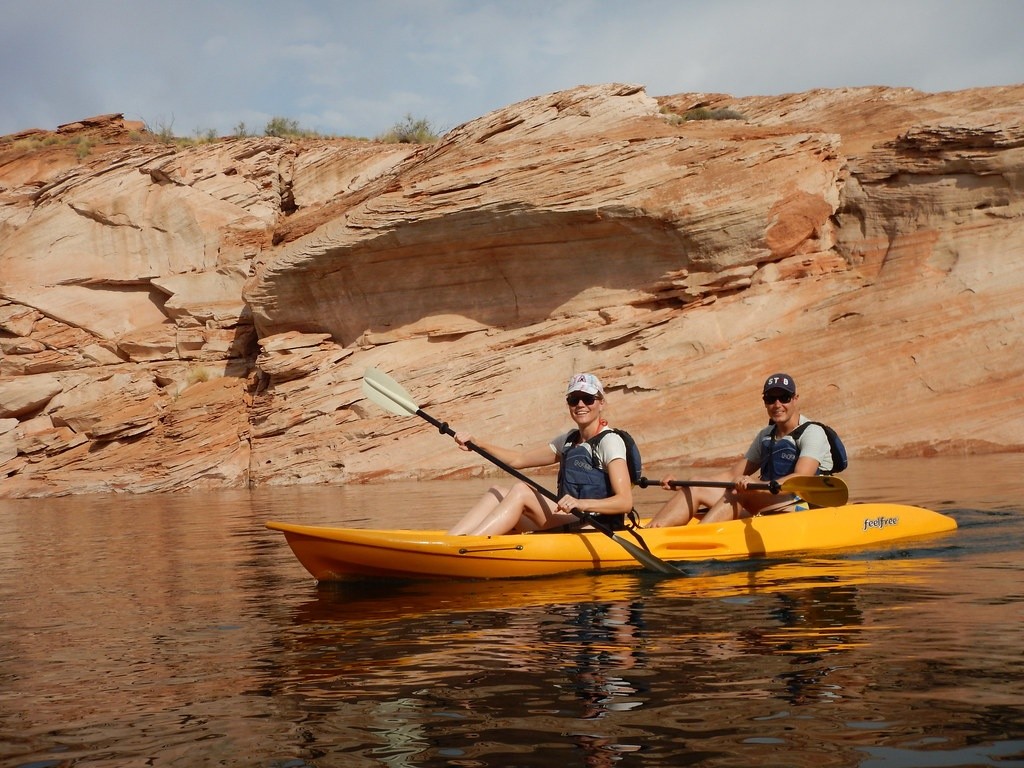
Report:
[360,370,691,579]
[631,475,850,509]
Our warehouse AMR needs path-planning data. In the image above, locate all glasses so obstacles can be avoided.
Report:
[762,391,795,404]
[566,393,601,407]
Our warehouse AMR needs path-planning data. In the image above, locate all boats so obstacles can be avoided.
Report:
[263,500,961,587]
[274,554,958,634]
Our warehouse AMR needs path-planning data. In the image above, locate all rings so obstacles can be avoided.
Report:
[565,503,570,507]
[744,479,747,481]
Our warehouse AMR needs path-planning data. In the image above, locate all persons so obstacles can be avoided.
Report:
[447,373,633,536]
[641,373,834,529]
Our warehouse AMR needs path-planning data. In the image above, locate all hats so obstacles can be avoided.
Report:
[566,374,605,400]
[763,373,796,394]
